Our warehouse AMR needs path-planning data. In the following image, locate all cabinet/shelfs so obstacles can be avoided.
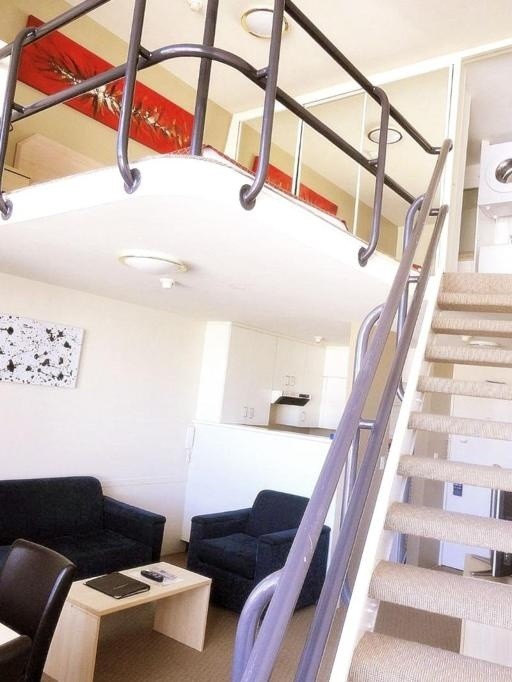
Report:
[277,343,325,428]
[320,345,354,430]
[273,331,307,397]
[197,321,277,426]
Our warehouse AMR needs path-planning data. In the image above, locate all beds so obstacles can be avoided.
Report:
[12,133,349,233]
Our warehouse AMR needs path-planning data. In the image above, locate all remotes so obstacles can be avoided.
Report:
[141,569,164,582]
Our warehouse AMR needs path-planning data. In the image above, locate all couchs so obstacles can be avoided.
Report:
[0,477,167,581]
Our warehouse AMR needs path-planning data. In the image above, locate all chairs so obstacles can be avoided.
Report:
[0,539,77,682]
[188,490,331,621]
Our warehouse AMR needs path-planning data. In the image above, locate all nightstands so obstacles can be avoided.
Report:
[1,164,31,193]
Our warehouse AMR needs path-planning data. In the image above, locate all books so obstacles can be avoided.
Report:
[82,572,151,600]
